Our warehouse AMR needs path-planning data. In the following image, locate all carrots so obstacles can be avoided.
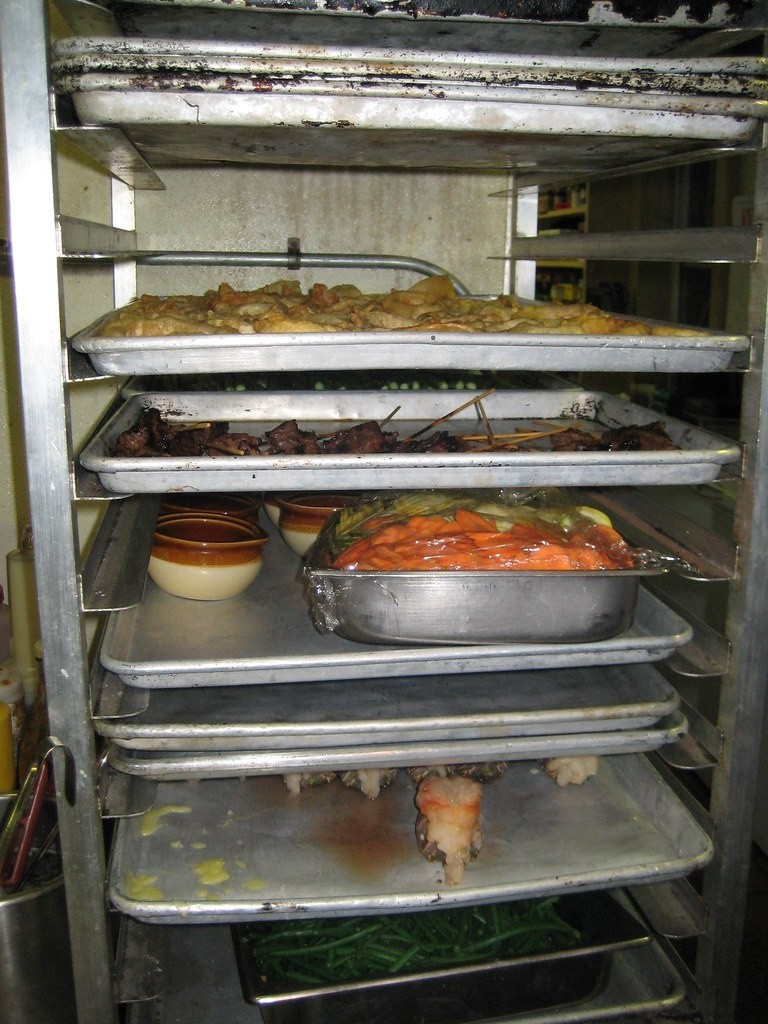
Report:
[331,509,635,571]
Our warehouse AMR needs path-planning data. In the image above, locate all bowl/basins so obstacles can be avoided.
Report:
[148,491,360,601]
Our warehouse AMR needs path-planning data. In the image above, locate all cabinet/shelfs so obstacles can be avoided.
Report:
[0,2,768,1024]
